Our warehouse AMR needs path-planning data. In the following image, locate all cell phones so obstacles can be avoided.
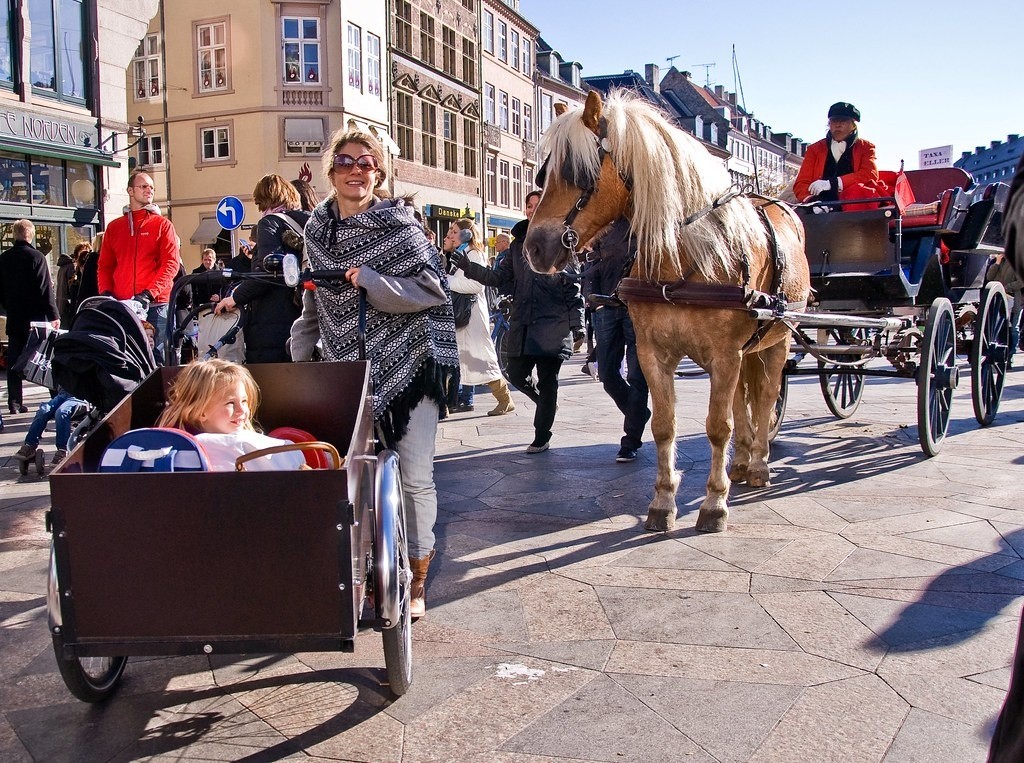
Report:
[239,239,252,252]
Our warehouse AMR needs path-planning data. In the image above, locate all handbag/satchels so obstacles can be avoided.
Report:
[10,321,70,391]
[451,291,476,327]
[198,304,247,365]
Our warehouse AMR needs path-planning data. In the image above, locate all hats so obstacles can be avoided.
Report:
[828,102,861,122]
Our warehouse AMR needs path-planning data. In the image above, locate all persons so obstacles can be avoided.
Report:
[140,319,164,367]
[290,179,319,213]
[793,101,887,299]
[215,173,312,364]
[172,225,259,363]
[15,389,94,468]
[293,133,460,617]
[159,357,261,433]
[57,232,105,333]
[581,226,653,461]
[97,173,180,368]
[450,189,583,452]
[0,219,61,414]
[427,220,516,415]
[489,233,512,374]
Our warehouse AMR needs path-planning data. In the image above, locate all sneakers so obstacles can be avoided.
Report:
[15,445,36,461]
[615,447,638,461]
[49,450,66,467]
[526,441,549,452]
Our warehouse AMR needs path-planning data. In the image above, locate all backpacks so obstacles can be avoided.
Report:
[97,428,211,472]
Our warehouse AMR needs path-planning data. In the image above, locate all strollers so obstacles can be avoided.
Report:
[50,298,248,456]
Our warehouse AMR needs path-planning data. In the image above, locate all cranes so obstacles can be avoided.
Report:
[665,54,682,69]
[691,62,717,87]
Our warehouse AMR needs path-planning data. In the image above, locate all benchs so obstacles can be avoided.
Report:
[889,167,980,233]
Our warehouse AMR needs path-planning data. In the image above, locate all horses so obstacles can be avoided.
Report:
[522,85,809,532]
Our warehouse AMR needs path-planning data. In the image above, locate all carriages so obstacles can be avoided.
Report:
[523,84,1011,534]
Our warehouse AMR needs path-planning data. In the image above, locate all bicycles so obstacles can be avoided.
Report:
[483,306,514,384]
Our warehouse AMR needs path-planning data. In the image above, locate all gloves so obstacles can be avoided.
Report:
[808,180,831,196]
[131,292,150,309]
[450,250,470,272]
[813,201,829,214]
[573,327,585,342]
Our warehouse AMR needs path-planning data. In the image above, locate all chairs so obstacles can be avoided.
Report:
[876,170,898,227]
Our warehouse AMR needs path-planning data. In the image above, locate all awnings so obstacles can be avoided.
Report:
[190,219,232,245]
[350,121,373,136]
[282,118,324,141]
[370,127,400,155]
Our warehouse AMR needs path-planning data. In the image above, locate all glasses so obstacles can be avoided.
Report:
[332,154,378,174]
[131,185,156,192]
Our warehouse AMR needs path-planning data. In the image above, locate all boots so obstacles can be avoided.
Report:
[407,549,435,617]
[587,340,595,355]
[488,378,516,416]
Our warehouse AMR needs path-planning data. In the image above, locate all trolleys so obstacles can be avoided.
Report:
[44,269,418,706]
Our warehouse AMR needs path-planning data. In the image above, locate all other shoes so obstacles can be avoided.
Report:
[588,362,598,381]
[582,365,599,377]
[451,401,475,412]
[10,406,28,415]
